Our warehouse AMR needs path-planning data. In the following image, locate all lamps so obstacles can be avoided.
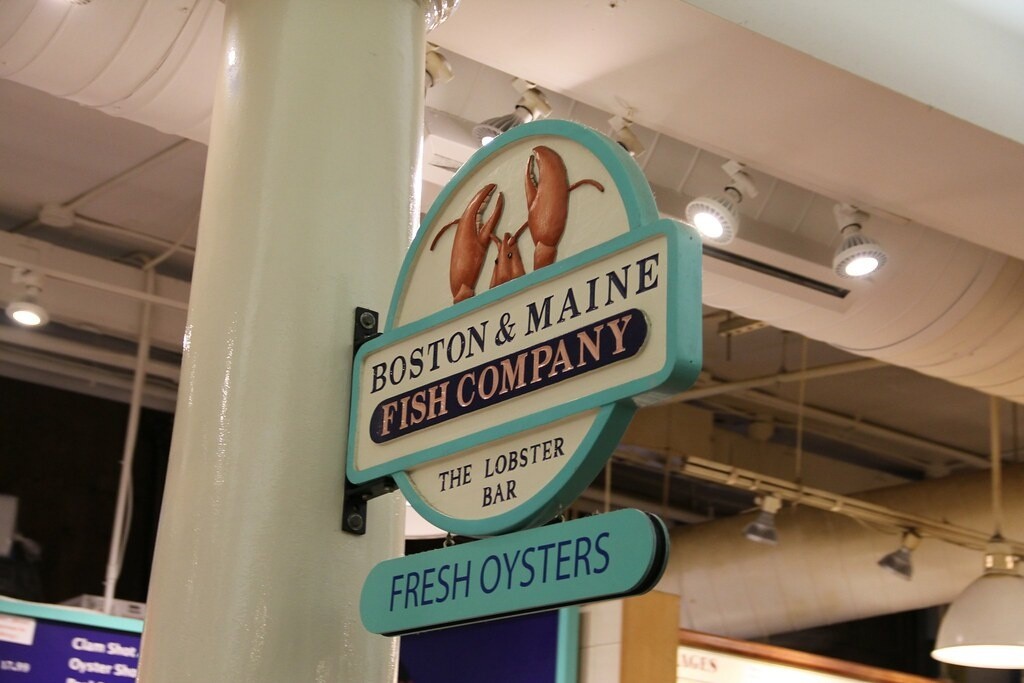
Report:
[472,78,554,150]
[686,159,760,248]
[3,268,49,329]
[744,495,782,547]
[878,528,923,583]
[930,395,1024,670]
[423,42,454,140]
[832,202,887,279]
